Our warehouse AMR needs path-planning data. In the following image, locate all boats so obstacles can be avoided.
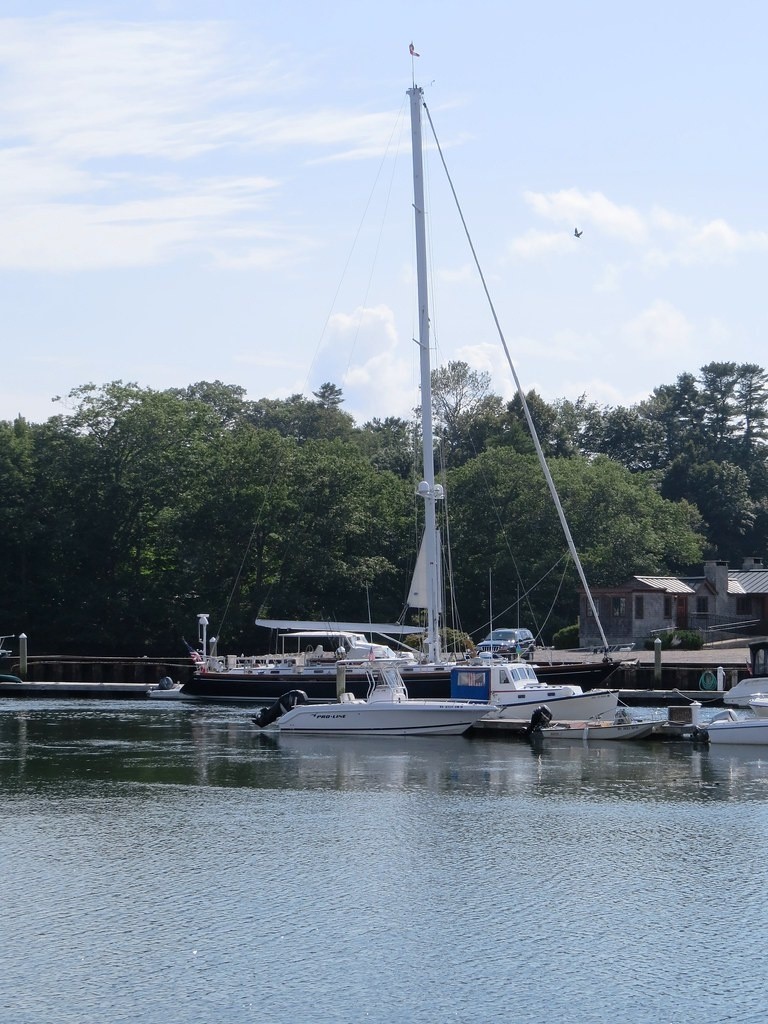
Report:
[724,678,768,708]
[262,691,502,740]
[749,698,768,716]
[450,665,618,719]
[521,706,666,743]
[691,712,767,744]
[149,677,185,701]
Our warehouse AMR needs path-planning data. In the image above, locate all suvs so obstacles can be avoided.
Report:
[478,627,535,662]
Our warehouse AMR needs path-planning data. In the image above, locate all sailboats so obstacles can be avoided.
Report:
[183,83,635,707]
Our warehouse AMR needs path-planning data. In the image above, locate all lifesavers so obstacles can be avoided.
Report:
[191,652,205,673]
